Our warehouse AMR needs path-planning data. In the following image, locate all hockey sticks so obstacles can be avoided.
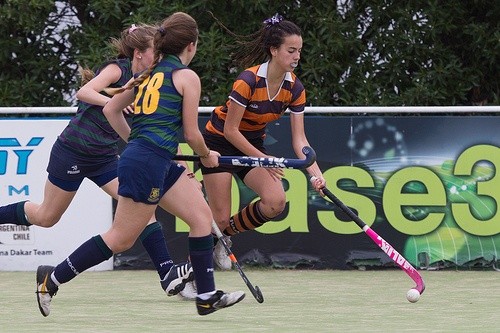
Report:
[307,175,426,295]
[212,218,265,303]
[172,146,317,169]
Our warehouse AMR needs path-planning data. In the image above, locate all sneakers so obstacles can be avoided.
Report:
[179,280,198,299]
[160,263,193,296]
[195,290,245,315]
[213,236,232,270]
[36,265,58,317]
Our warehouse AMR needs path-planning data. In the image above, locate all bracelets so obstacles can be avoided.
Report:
[198,149,211,158]
[187,172,196,179]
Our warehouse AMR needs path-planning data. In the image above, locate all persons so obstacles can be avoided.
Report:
[35,12,246,317]
[179,14,327,300]
[1,23,205,297]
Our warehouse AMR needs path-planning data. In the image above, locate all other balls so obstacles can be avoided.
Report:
[406,289,420,303]
